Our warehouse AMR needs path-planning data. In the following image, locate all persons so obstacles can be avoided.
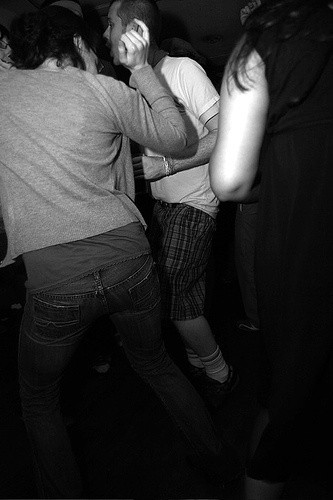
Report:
[0,0,240,500]
[210,0,333,500]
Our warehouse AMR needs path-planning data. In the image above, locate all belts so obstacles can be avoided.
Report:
[159,201,182,211]
[236,202,257,215]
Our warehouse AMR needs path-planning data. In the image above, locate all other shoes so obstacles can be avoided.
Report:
[176,356,205,391]
[237,319,259,332]
[95,350,112,374]
[200,359,242,412]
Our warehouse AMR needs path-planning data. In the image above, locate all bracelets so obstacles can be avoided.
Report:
[160,157,173,176]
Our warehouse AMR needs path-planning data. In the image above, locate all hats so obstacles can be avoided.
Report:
[160,38,208,64]
